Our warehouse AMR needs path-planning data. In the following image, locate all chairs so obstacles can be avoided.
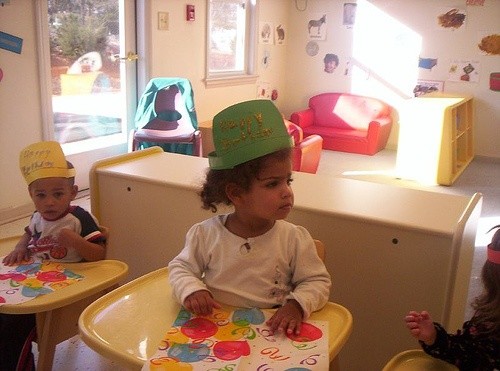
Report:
[1,226,129,371]
[78,239,353,371]
[381,350,459,371]
[128,77,202,157]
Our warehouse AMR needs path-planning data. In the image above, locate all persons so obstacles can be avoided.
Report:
[406,228,500,371]
[168,100,331,337]
[2,142,105,266]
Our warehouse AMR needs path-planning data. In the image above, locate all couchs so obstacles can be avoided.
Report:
[284,120,323,174]
[291,93,393,156]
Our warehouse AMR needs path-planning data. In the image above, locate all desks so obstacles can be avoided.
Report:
[200,120,216,157]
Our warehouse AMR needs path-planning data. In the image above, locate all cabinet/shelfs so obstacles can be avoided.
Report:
[90,146,484,370]
[397,92,476,186]
